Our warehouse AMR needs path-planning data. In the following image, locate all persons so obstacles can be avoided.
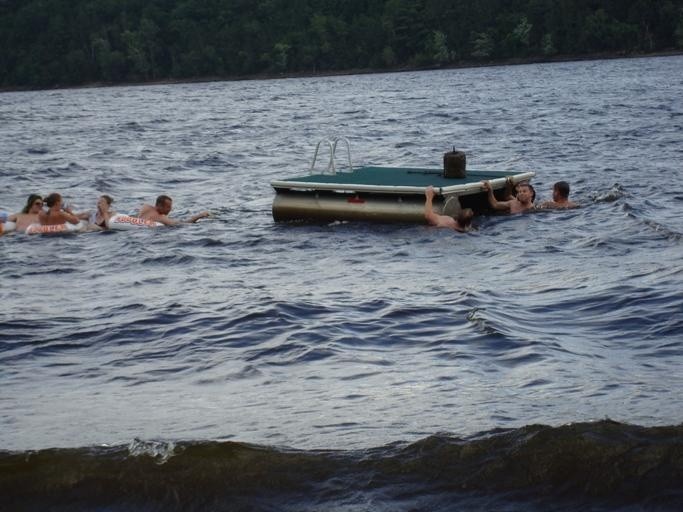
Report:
[7,194,45,233]
[138,196,210,227]
[38,193,80,225]
[425,178,577,231]
[76,196,114,231]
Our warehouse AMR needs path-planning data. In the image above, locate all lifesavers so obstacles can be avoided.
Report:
[109,214,165,230]
[0,212,17,233]
[26,221,84,235]
